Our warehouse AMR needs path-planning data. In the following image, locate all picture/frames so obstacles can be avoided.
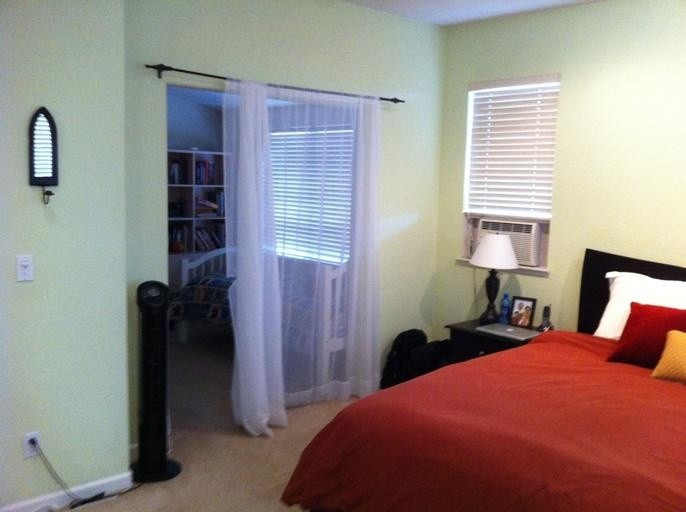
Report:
[510,294,536,329]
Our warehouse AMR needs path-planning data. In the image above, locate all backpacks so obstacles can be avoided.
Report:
[379,328,452,389]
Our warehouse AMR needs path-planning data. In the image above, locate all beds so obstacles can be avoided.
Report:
[291,244,684,512]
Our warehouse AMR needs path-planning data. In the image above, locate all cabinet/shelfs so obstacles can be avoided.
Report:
[168,148,234,295]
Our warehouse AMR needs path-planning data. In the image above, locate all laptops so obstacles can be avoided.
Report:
[475,322,544,341]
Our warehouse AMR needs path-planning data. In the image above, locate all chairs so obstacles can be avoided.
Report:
[177,243,238,349]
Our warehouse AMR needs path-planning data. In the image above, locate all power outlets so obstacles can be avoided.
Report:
[23,431,42,460]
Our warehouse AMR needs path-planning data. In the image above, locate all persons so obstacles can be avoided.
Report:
[510,311,519,325]
[518,306,530,327]
[513,301,524,313]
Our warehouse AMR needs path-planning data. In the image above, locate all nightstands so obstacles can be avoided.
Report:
[445,316,546,361]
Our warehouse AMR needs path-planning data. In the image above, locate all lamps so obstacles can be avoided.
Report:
[468,234,521,323]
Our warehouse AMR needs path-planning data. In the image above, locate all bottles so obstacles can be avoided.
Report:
[499,293,513,324]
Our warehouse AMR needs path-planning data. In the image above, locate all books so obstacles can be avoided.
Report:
[167,159,225,255]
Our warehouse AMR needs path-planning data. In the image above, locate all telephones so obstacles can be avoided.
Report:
[538,304,552,331]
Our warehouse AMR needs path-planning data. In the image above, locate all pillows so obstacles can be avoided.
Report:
[594,266,684,386]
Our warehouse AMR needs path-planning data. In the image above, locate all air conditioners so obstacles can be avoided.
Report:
[472,219,541,268]
